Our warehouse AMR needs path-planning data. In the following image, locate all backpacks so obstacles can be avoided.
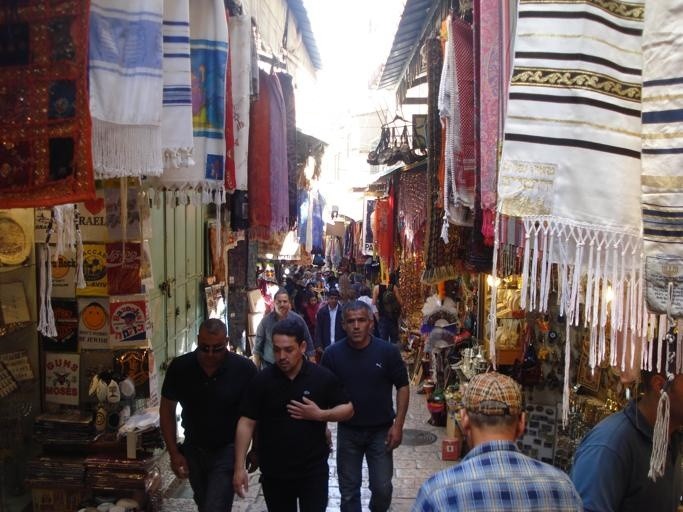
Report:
[383,284,400,313]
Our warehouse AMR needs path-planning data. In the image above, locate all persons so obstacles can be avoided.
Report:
[232,320,354,512]
[160,319,257,512]
[319,300,409,512]
[409,371,583,512]
[252,263,404,371]
[571,332,683,512]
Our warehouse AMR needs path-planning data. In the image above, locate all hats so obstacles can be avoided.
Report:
[462,370,526,416]
